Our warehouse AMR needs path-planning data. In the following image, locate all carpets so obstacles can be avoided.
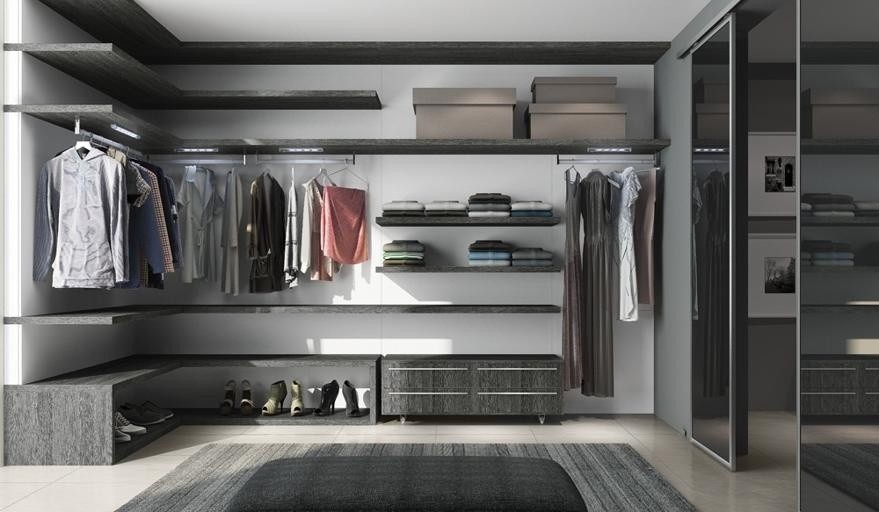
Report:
[113,441,697,510]
[802,441,879,512]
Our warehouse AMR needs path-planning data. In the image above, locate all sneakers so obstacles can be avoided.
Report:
[142,402,174,419]
[114,426,130,443]
[114,411,146,434]
[118,404,165,425]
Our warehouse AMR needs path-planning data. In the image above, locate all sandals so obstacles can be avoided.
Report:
[240,379,254,414]
[220,380,235,415]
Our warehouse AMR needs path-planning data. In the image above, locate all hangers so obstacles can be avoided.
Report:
[289,157,369,190]
[74,131,109,153]
[564,163,661,189]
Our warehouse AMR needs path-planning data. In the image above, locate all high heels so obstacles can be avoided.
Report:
[314,381,339,416]
[262,381,287,415]
[290,382,304,415]
[343,380,360,417]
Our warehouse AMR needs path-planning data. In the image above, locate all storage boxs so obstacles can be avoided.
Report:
[691,79,729,146]
[411,77,629,139]
[800,89,877,142]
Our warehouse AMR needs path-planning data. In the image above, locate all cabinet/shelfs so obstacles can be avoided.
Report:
[3,0,182,156]
[381,352,564,426]
[801,353,878,417]
[799,43,878,154]
[179,40,671,157]
[799,215,877,311]
[3,304,179,392]
[376,213,565,314]
[171,301,383,368]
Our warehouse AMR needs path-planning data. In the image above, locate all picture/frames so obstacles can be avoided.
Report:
[745,131,798,221]
[746,230,797,323]
